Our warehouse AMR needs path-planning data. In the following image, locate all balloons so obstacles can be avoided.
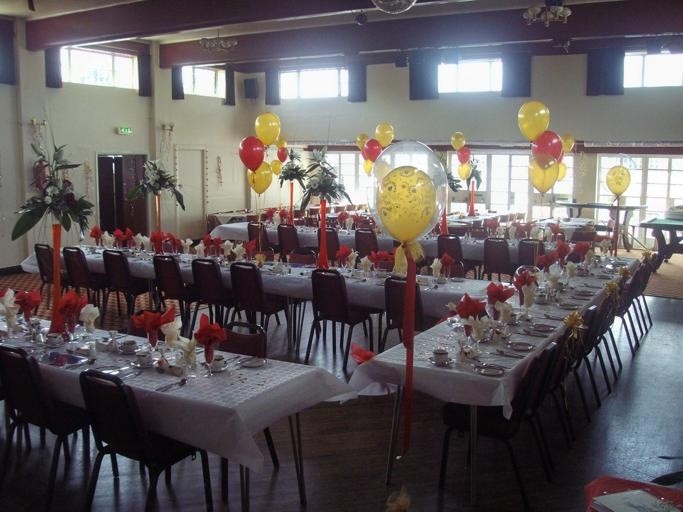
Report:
[557,162,567,182]
[451,132,466,151]
[363,158,373,175]
[277,148,288,163]
[361,148,368,160]
[239,135,265,174]
[255,112,281,149]
[517,99,551,142]
[276,137,287,148]
[364,139,382,163]
[374,121,395,149]
[246,161,273,198]
[531,130,564,170]
[458,162,472,181]
[560,133,575,151]
[377,167,437,245]
[271,159,282,176]
[367,139,449,246]
[457,146,471,164]
[606,166,631,198]
[356,133,368,150]
[599,153,637,200]
[528,152,559,198]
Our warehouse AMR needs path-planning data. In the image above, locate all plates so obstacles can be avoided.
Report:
[129,360,156,368]
[210,363,229,372]
[118,348,141,353]
[533,325,555,331]
[47,344,60,347]
[473,366,505,376]
[427,357,455,364]
[451,278,463,282]
[508,342,534,351]
[238,356,267,369]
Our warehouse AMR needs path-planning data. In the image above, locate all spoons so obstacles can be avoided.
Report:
[426,285,439,294]
[155,378,186,391]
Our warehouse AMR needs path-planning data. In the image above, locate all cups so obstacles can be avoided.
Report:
[213,355,224,367]
[433,269,440,285]
[471,326,484,354]
[255,254,264,270]
[335,260,338,267]
[183,246,190,254]
[108,329,118,344]
[500,311,511,337]
[136,351,152,363]
[148,332,158,351]
[47,333,57,344]
[135,242,141,252]
[561,303,578,309]
[328,260,331,266]
[375,268,387,286]
[186,347,197,379]
[441,274,445,282]
[444,264,450,281]
[123,340,138,352]
[287,265,292,275]
[164,335,177,361]
[433,349,449,363]
[341,257,347,271]
[447,317,457,325]
[453,323,460,332]
[463,323,472,349]
[524,293,534,320]
[204,345,215,379]
[366,271,375,286]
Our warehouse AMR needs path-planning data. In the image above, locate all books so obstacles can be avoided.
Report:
[590,488,683,512]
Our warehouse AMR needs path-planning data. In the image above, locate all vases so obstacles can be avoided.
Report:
[468,181,474,215]
[154,190,162,195]
[50,225,64,332]
[155,195,161,228]
[319,200,328,269]
[320,191,327,195]
[443,211,448,235]
[289,182,294,225]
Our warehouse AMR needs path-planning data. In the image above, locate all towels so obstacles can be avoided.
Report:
[520,282,537,308]
[546,272,559,287]
[15,288,42,320]
[167,233,181,252]
[143,235,151,250]
[336,245,352,266]
[1,288,21,327]
[345,217,354,232]
[151,232,167,254]
[265,208,277,221]
[272,211,282,225]
[585,250,592,265]
[244,239,256,257]
[515,223,523,240]
[513,271,534,306]
[529,227,540,240]
[548,223,559,235]
[573,241,589,261]
[194,240,206,258]
[203,234,212,256]
[232,243,246,258]
[456,293,485,336]
[194,313,227,364]
[524,223,532,237]
[494,300,512,323]
[1,288,8,297]
[179,332,198,363]
[556,243,570,265]
[181,238,194,253]
[535,251,558,273]
[470,313,484,342]
[484,220,497,234]
[429,257,442,278]
[161,319,179,346]
[102,230,116,249]
[486,282,515,320]
[279,208,288,221]
[508,226,516,239]
[114,229,127,248]
[59,289,89,333]
[80,303,100,328]
[599,238,611,253]
[132,303,175,349]
[338,212,348,226]
[132,233,143,249]
[126,228,133,248]
[219,240,234,255]
[440,252,454,277]
[212,237,222,256]
[348,248,359,266]
[360,256,373,274]
[545,226,552,241]
[565,260,576,278]
[354,216,363,228]
[369,251,388,269]
[497,226,502,236]
[90,226,102,246]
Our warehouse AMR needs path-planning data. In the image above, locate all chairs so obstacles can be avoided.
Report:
[519,238,542,269]
[335,206,345,212]
[247,214,259,222]
[305,269,374,371]
[236,209,247,213]
[12,290,38,315]
[505,227,525,239]
[317,228,339,266]
[596,226,606,242]
[488,211,497,213]
[308,217,317,225]
[164,322,280,503]
[294,210,304,217]
[80,371,212,512]
[278,224,297,262]
[500,214,508,234]
[361,217,374,229]
[455,212,460,215]
[152,256,186,323]
[437,342,558,511]
[74,310,165,438]
[510,213,515,223]
[583,287,615,409]
[155,239,183,253]
[190,259,242,338]
[103,250,157,317]
[416,256,437,276]
[492,217,499,235]
[0,346,91,490]
[261,213,271,220]
[434,225,440,233]
[615,268,641,358]
[438,235,476,279]
[517,212,525,222]
[204,247,235,259]
[335,248,359,269]
[562,305,597,442]
[599,276,624,381]
[572,230,596,245]
[247,222,268,251]
[326,207,331,214]
[640,257,659,325]
[447,213,453,216]
[481,238,509,282]
[473,221,481,228]
[289,248,316,264]
[553,230,564,242]
[252,247,273,260]
[628,260,652,336]
[371,254,395,271]
[348,205,355,210]
[207,214,216,232]
[380,278,424,351]
[607,227,613,240]
[63,247,103,306]
[223,211,234,213]
[484,219,493,235]
[225,262,290,358]
[310,208,320,218]
[471,228,485,238]
[359,204,367,210]
[35,245,69,315]
[526,325,573,472]
[355,229,378,268]
[113,236,136,248]
[325,218,338,227]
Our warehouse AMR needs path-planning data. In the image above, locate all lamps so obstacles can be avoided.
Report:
[520,0,572,28]
[199,28,237,53]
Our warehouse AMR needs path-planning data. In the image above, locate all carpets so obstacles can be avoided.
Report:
[595,248,683,300]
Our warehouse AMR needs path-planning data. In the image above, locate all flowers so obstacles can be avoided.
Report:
[465,159,482,199]
[279,149,307,190]
[11,142,94,241]
[301,147,352,211]
[124,160,185,210]
[438,154,462,192]
